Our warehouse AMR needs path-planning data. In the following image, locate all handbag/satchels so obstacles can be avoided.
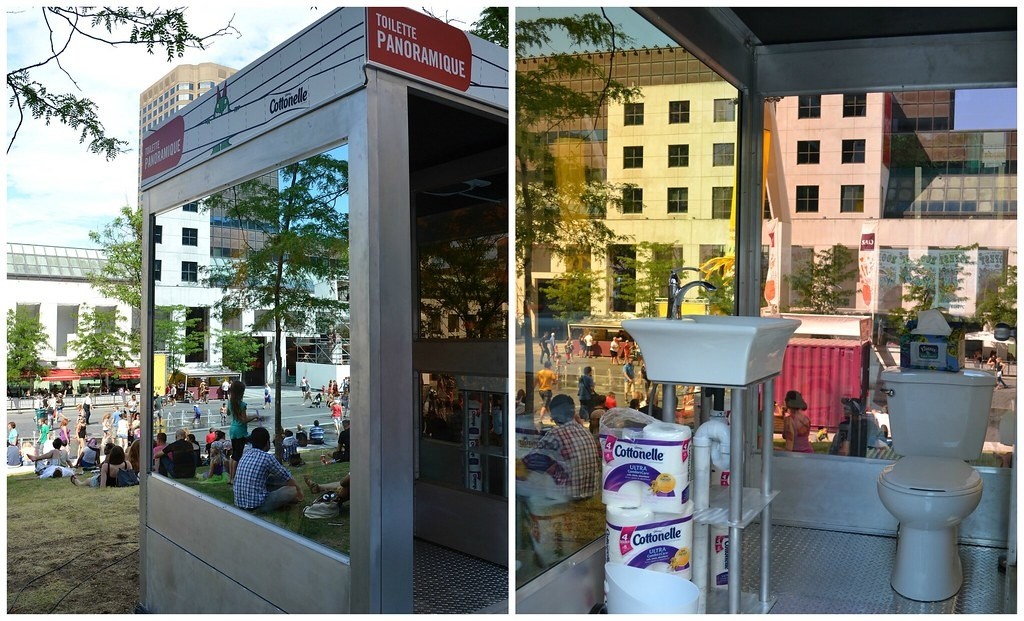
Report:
[289,453,300,466]
[589,389,600,406]
[57,398,64,408]
[118,468,138,487]
[303,500,339,519]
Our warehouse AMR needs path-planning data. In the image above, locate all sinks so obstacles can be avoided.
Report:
[621,315,802,385]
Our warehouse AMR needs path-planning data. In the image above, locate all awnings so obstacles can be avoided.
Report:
[7,366,143,383]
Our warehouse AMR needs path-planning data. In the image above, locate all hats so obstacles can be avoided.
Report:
[841,396,861,417]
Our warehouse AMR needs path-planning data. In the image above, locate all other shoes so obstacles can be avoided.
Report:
[624,399,629,404]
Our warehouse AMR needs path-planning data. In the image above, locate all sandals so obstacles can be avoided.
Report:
[303,475,320,495]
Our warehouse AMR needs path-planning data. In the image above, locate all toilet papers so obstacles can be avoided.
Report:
[709,465,732,592]
[601,421,694,568]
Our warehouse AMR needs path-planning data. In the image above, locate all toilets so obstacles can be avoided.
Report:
[876,366,998,604]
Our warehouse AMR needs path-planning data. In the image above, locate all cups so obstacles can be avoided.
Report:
[320,455,325,465]
[849,414,866,457]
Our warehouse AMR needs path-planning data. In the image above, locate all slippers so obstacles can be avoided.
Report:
[71,475,77,485]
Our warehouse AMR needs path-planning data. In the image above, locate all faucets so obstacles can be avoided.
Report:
[667,266,717,319]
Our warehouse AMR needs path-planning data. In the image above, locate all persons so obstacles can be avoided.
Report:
[986,351,997,371]
[835,397,862,457]
[781,390,814,454]
[149,325,698,510]
[6,380,143,493]
[993,357,1008,392]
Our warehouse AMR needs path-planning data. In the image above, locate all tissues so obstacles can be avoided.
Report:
[899,308,967,374]
